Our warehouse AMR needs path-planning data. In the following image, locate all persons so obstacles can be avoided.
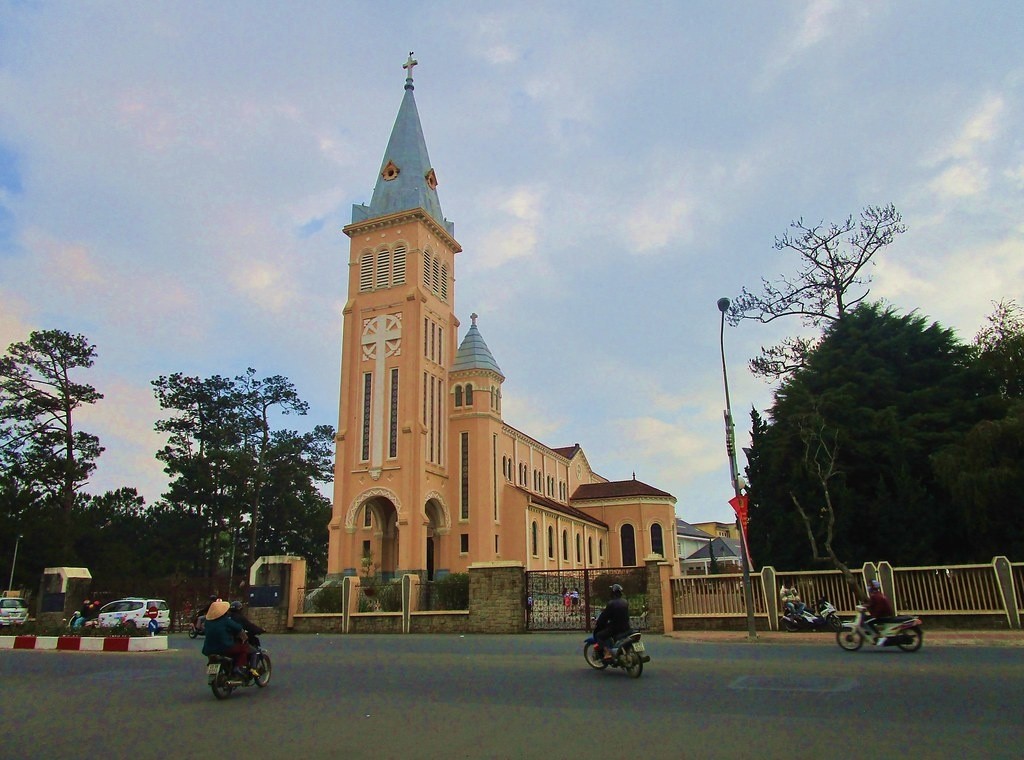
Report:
[780,577,805,624]
[595,584,630,660]
[73,600,100,630]
[183,599,192,617]
[862,580,894,646]
[527,592,532,622]
[202,600,264,679]
[193,595,216,630]
[563,587,579,616]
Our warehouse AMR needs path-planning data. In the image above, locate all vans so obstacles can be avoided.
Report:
[0,597,30,628]
[98,597,171,635]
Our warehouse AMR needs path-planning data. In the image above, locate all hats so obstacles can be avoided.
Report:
[205,601,230,620]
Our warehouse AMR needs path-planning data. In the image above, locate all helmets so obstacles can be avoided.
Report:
[609,584,622,594]
[231,601,242,611]
[868,580,881,592]
[210,595,217,601]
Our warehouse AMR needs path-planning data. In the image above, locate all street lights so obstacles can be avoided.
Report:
[8,533,24,590]
[716,297,758,640]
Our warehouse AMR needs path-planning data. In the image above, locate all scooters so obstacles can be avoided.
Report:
[778,593,843,633]
[65,609,104,636]
[189,608,209,638]
[835,600,924,653]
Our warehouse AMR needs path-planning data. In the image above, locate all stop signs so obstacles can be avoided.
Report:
[148,607,158,619]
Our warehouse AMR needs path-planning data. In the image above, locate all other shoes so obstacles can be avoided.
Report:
[250,669,259,676]
[877,637,887,645]
[232,667,243,675]
[603,653,612,659]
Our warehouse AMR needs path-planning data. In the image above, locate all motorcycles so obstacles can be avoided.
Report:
[583,616,650,678]
[206,629,272,700]
